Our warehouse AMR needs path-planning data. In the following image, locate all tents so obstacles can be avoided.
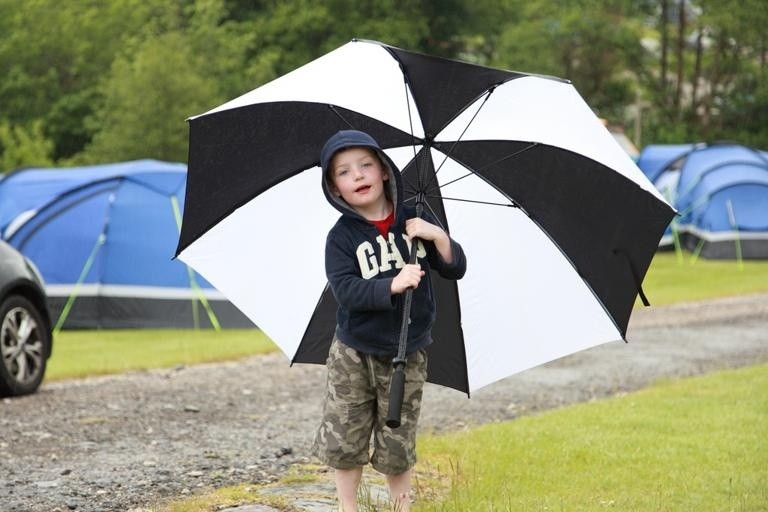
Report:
[630,141,767,260]
[1,171,257,333]
[1,155,165,236]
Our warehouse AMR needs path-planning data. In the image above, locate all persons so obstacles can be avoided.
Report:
[311,129,469,510]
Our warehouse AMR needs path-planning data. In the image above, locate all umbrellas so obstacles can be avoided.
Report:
[170,31,685,402]
